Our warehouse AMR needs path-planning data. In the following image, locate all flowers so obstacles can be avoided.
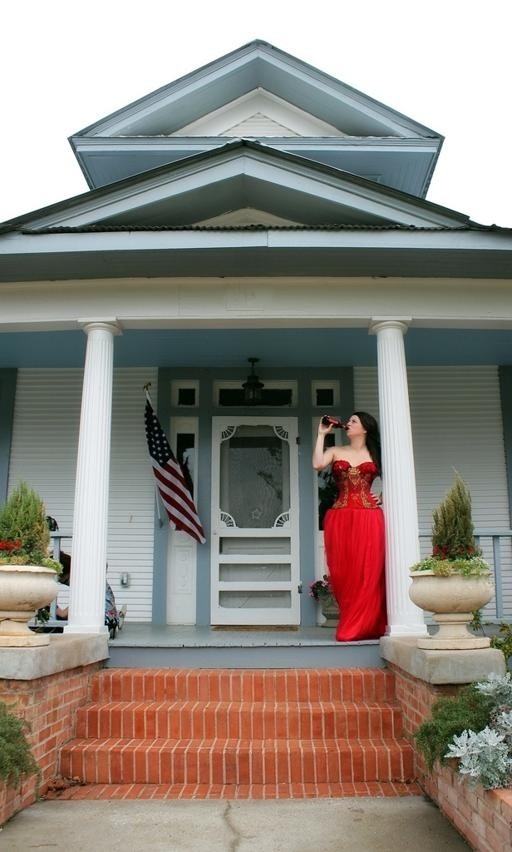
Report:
[307,574,338,608]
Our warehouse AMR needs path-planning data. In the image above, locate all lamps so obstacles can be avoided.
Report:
[242,358,264,401]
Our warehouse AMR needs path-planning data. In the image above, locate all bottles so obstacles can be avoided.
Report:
[322,415,349,431]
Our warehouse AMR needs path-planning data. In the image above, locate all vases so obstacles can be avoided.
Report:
[320,594,341,628]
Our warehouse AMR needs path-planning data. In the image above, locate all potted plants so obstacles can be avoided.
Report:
[0,480,63,648]
[409,464,495,650]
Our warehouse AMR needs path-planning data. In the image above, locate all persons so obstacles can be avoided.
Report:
[312,412,386,643]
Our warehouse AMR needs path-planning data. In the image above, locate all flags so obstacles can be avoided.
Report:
[144,396,206,544]
